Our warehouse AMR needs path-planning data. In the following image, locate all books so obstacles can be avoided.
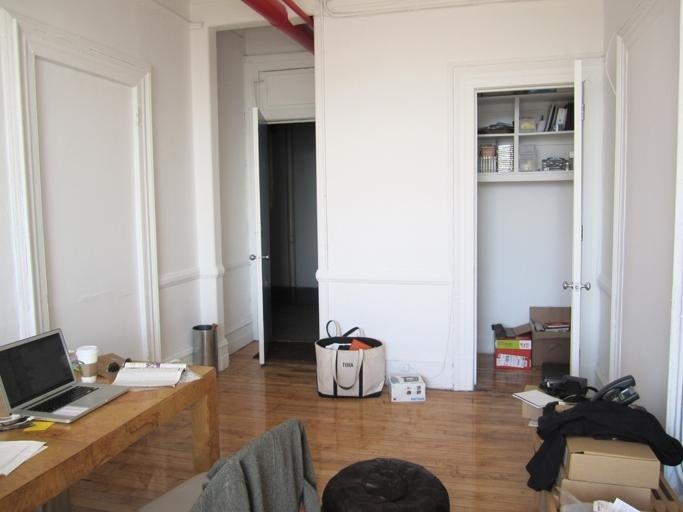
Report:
[111,360,187,388]
[496,143,514,172]
[543,105,568,132]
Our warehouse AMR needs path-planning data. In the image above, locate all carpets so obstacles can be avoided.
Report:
[251,340,318,365]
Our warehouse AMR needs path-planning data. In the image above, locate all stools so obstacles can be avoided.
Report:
[320,456,450,511]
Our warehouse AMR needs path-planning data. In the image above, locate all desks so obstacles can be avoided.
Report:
[0,362,220,511]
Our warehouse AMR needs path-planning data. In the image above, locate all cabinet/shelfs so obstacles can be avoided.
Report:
[476,90,574,183]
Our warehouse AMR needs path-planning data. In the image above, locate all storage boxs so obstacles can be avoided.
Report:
[528,305,569,366]
[388,374,426,403]
[521,383,662,512]
[494,334,532,370]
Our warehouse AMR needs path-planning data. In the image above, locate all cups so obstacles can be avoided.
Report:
[75,344,98,385]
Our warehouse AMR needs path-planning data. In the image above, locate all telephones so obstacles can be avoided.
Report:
[591,375,640,406]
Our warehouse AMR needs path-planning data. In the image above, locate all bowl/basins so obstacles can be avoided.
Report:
[480,147,497,156]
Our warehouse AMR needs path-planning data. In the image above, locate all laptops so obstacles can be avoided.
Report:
[0,327,129,424]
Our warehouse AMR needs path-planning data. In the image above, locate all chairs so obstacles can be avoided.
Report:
[135,416,315,511]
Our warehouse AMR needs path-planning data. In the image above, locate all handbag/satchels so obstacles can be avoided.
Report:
[315,320,386,398]
